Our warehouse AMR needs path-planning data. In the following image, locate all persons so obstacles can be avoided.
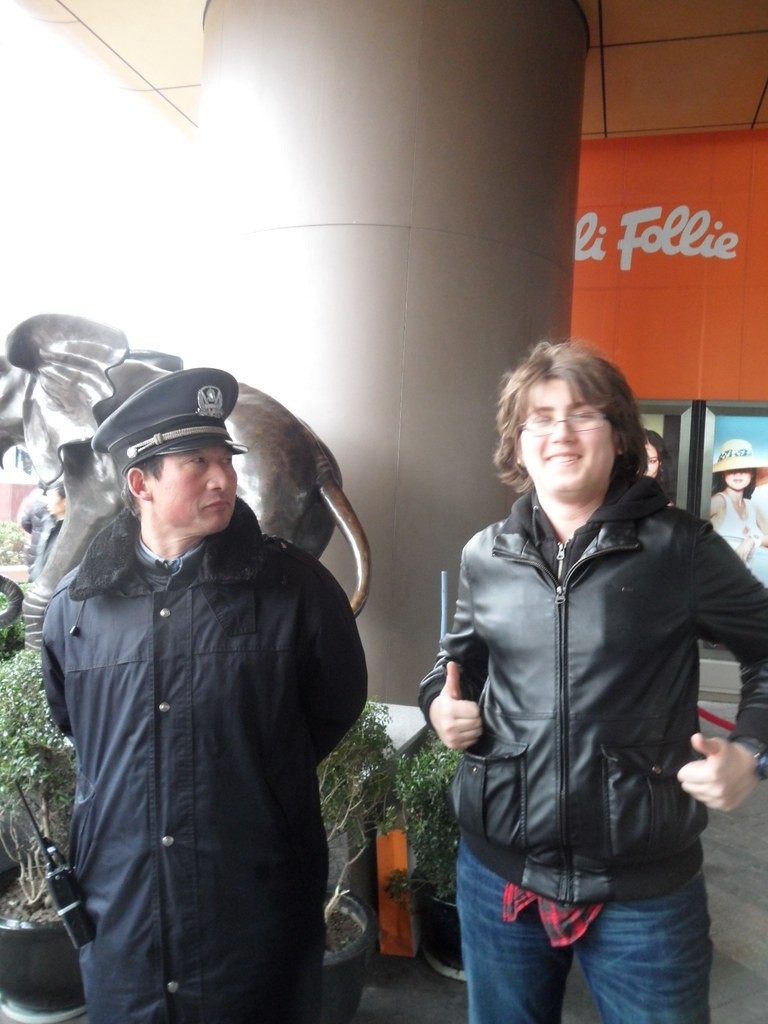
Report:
[27,482,67,586]
[418,340,768,1024]
[710,439,768,588]
[635,429,675,506]
[42,366,369,1024]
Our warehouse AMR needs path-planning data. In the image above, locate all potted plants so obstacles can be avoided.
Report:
[314,697,402,1024]
[391,728,470,984]
[0,648,86,1023]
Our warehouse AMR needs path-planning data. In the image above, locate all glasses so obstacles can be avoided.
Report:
[513,411,605,437]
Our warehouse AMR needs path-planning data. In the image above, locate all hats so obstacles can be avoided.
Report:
[89,366,251,476]
[712,438,768,473]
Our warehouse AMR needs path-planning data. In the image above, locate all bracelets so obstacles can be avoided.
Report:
[752,751,768,781]
